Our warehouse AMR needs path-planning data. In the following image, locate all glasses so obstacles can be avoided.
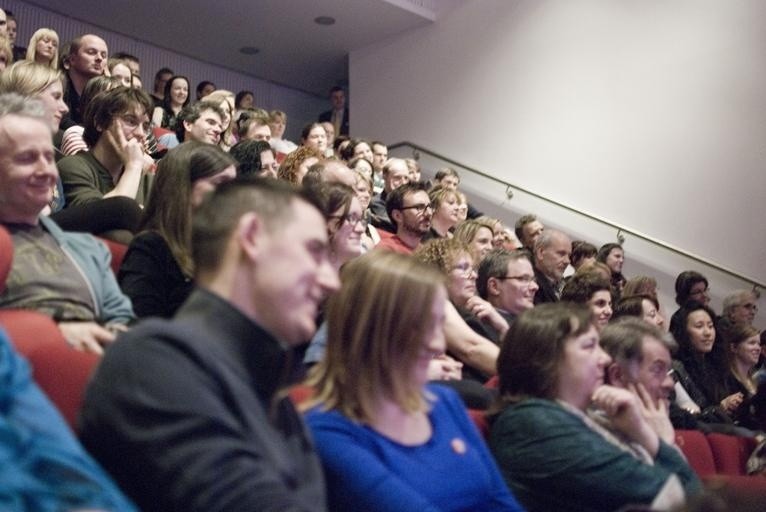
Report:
[395,202,436,213]
[495,273,540,284]
[112,110,154,133]
[447,262,480,280]
[326,211,371,229]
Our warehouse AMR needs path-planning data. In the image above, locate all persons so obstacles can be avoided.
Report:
[0,5,349,512]
[305,137,766,510]
